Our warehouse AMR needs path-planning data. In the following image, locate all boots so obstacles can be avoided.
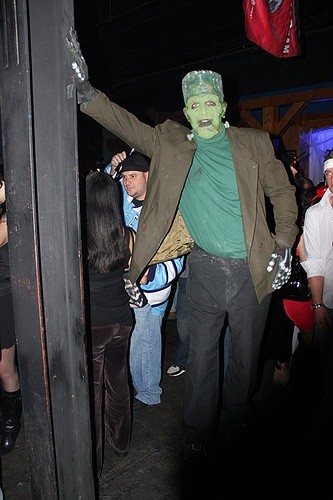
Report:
[272,360,289,384]
[0,387,21,456]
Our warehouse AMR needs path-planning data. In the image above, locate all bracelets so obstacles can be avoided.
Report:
[313,303,323,311]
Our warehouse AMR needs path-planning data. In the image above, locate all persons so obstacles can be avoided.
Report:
[104,150,184,414]
[253,148,333,443]
[84,173,144,477]
[0,179,23,455]
[65,27,300,470]
[166,255,189,376]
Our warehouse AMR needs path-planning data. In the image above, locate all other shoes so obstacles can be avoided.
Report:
[179,442,212,483]
[104,432,128,457]
[224,423,250,449]
[166,363,185,377]
[131,397,161,410]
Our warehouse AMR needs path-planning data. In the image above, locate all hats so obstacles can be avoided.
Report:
[181,69,224,103]
[114,150,149,173]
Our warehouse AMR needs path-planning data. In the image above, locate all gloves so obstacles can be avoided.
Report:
[64,26,101,104]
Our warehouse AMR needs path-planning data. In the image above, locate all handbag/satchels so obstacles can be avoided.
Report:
[279,255,316,334]
